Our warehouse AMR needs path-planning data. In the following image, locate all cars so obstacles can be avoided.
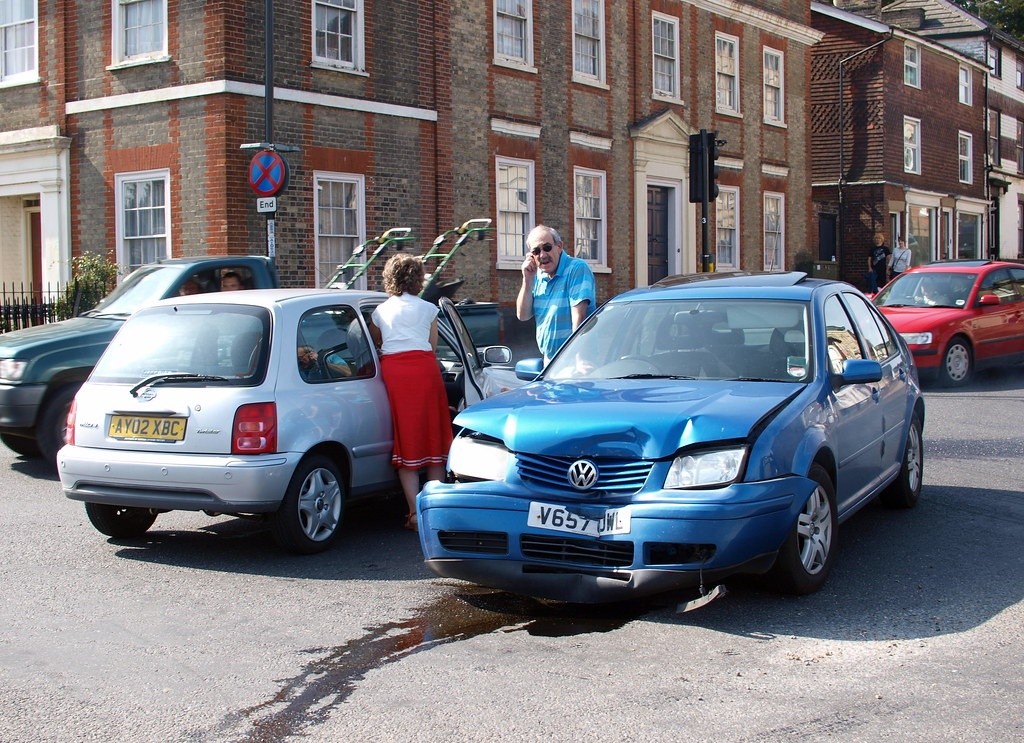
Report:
[414,270,926,602]
[862,258,1024,388]
[56,287,513,553]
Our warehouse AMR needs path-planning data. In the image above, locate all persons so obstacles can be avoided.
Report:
[914,278,951,305]
[517,225,596,380]
[886,236,911,280]
[297,344,351,379]
[868,234,892,295]
[179,276,199,296]
[221,271,245,292]
[368,252,453,532]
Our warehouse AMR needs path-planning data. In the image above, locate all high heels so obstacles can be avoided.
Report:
[404,512,419,532]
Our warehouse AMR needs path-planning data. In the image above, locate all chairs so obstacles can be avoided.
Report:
[647,313,720,377]
[748,320,805,378]
[215,331,261,376]
[308,327,356,380]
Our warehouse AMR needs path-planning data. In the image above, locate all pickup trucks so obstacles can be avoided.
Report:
[1,251,505,477]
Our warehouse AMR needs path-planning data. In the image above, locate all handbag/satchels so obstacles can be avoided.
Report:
[888,268,893,276]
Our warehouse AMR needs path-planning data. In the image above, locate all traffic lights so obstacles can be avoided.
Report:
[687,131,721,205]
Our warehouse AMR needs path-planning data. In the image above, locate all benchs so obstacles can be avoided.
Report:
[708,322,805,376]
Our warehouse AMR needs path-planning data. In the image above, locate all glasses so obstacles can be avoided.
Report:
[297,352,306,360]
[530,242,554,256]
